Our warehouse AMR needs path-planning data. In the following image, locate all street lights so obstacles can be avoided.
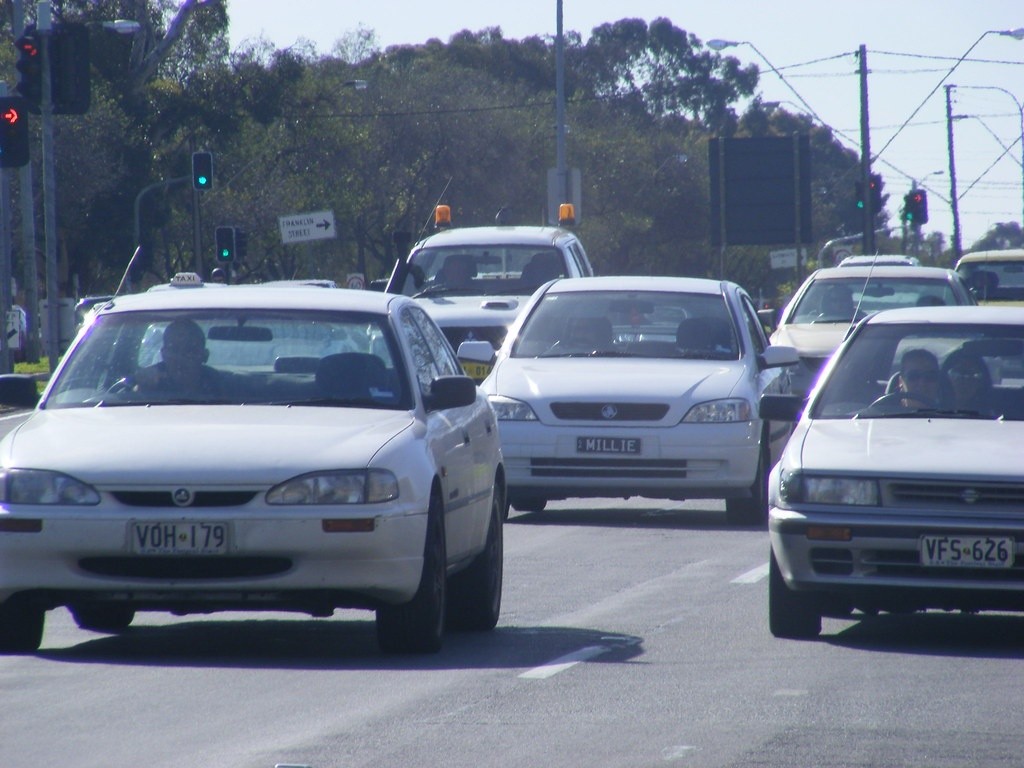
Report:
[764,101,963,261]
[912,171,945,250]
[705,29,1024,256]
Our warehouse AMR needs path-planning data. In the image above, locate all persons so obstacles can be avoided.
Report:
[125,319,247,408]
[821,284,867,321]
[211,268,224,283]
[900,349,939,408]
[941,347,993,419]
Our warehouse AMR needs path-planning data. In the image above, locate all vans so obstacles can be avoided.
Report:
[367,226,595,387]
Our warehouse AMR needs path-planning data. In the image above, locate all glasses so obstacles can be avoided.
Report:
[905,370,937,382]
[162,339,200,355]
[951,372,984,383]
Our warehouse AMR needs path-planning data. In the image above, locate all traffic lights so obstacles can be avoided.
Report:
[215,226,236,263]
[870,176,881,206]
[0,97,30,165]
[16,22,42,115]
[191,151,213,191]
[910,189,927,225]
[904,195,913,220]
[855,182,865,209]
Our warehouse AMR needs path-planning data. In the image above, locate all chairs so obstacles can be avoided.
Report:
[565,317,612,355]
[519,254,560,291]
[317,353,385,403]
[677,316,731,356]
[817,288,866,322]
[917,295,945,306]
[973,271,998,299]
[434,255,477,293]
[886,369,953,413]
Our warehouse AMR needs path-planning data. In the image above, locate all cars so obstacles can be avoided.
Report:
[755,264,1003,386]
[838,254,922,315]
[75,273,358,366]
[2,284,509,657]
[456,276,801,523]
[759,305,1024,641]
[944,249,1024,305]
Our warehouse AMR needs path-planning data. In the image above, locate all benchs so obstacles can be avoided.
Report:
[979,387,1024,420]
[612,333,677,355]
[221,371,317,401]
